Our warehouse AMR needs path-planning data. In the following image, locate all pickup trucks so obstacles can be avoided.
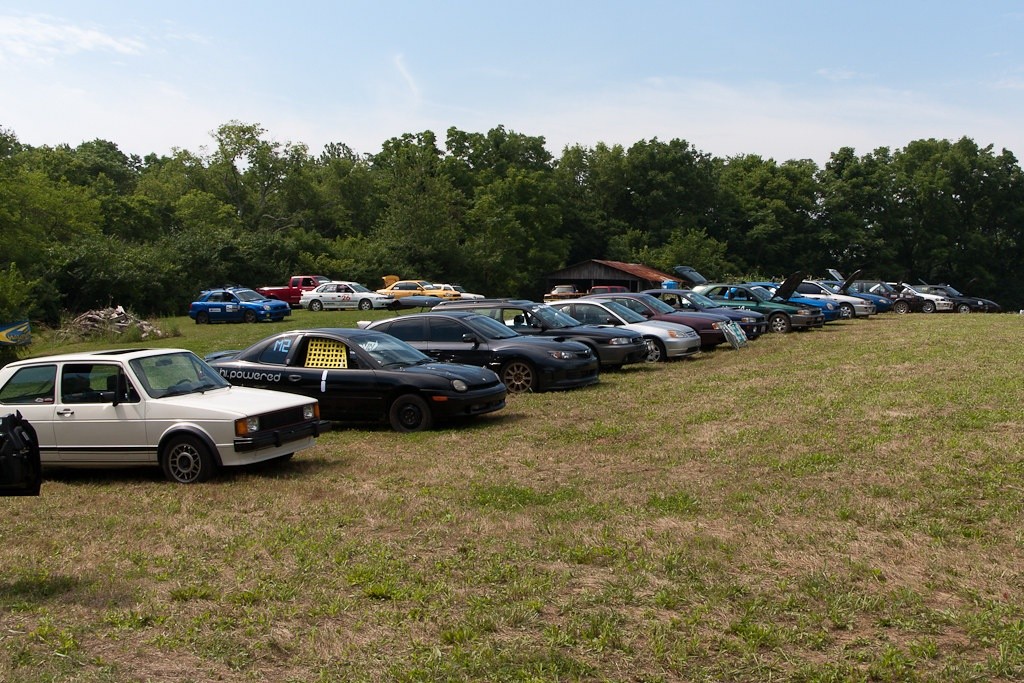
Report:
[255,276,331,304]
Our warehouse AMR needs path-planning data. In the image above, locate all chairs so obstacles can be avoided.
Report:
[670,298,676,305]
[99,374,128,402]
[738,291,746,301]
[665,300,671,306]
[617,300,626,307]
[628,301,636,311]
[46,372,99,402]
[731,292,735,300]
[514,314,524,327]
[586,309,601,322]
[576,310,584,321]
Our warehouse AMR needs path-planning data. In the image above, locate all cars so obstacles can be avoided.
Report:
[0,347,323,485]
[672,265,709,288]
[299,274,486,312]
[682,267,1003,340]
[356,311,601,394]
[422,284,770,412]
[197,328,507,435]
[188,287,292,325]
